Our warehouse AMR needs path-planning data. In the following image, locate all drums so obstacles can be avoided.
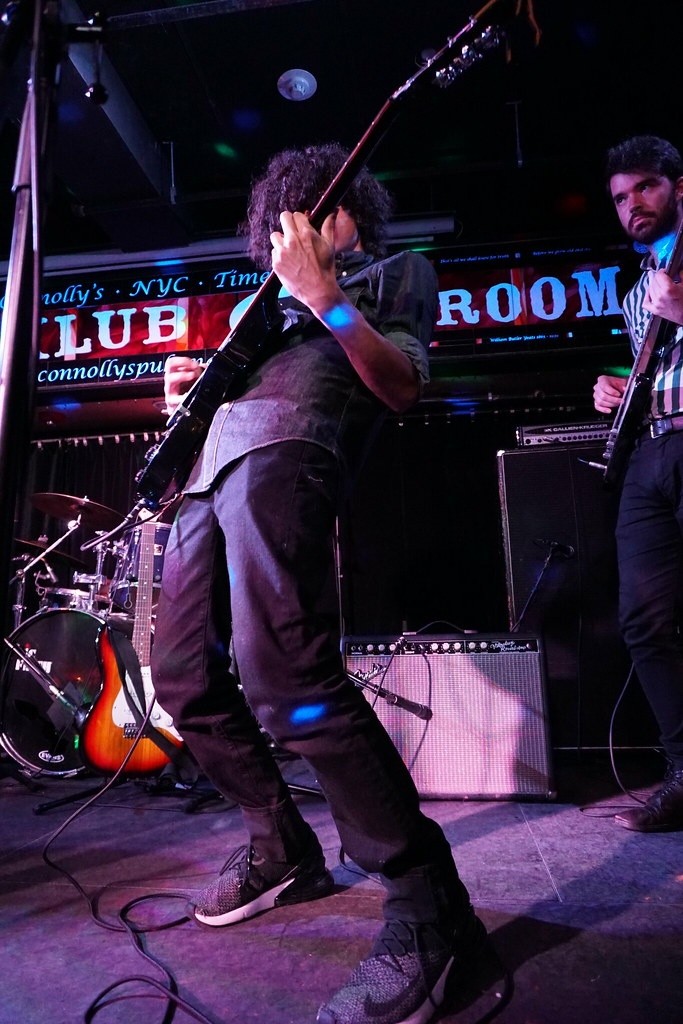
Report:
[106,520,174,616]
[0,606,157,777]
[38,586,124,622]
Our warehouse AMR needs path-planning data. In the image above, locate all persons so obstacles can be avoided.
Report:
[149,141,485,1022]
[592,136,683,832]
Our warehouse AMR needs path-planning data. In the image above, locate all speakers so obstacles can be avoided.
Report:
[341,630,581,804]
[495,445,665,749]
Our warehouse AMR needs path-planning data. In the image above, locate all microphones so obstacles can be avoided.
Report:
[44,562,58,584]
[533,539,574,558]
[126,498,147,520]
[384,691,432,720]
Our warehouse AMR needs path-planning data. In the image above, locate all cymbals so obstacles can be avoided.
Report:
[29,492,126,526]
[12,537,88,567]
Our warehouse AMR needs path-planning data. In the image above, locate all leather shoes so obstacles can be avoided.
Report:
[614,779,682,832]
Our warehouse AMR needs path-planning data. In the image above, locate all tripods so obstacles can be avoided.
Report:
[34,770,327,818]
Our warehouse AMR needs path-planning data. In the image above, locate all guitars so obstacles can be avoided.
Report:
[599,219,683,481]
[76,520,186,773]
[132,0,511,513]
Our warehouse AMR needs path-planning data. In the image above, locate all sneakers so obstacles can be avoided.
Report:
[185,843,336,928]
[316,918,511,1023]
